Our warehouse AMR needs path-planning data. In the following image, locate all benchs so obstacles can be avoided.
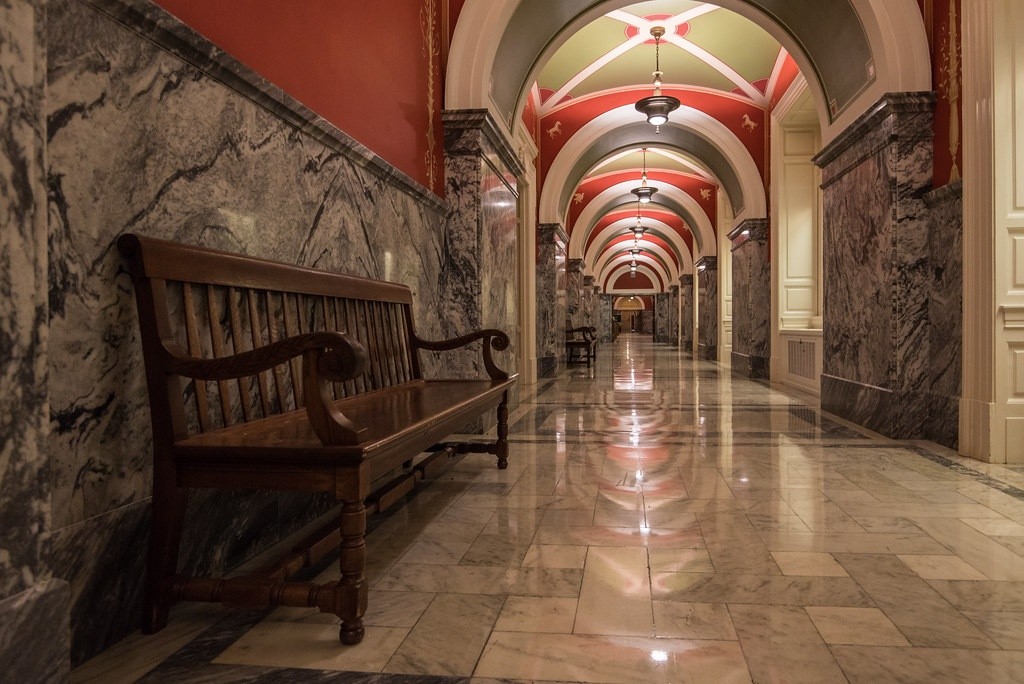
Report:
[116,233,516,646]
[566,326,597,368]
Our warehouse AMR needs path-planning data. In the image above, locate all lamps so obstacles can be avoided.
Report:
[627,238,645,259]
[631,147,658,206]
[629,205,650,240]
[629,257,640,272]
[635,27,681,134]
